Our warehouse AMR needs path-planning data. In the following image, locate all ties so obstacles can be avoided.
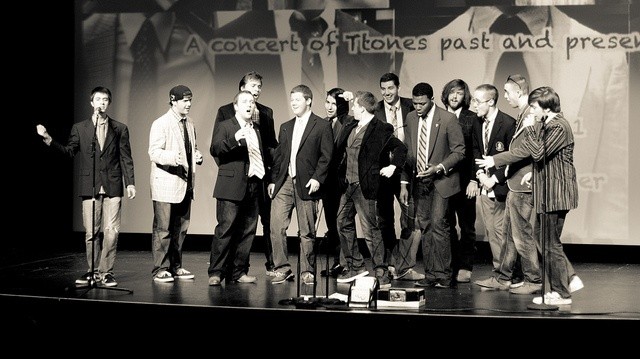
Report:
[417,117,427,179]
[289,18,327,118]
[391,106,398,138]
[329,116,336,129]
[484,120,491,156]
[491,16,532,122]
[128,23,159,178]
[180,119,189,160]
[246,123,265,179]
[99,118,106,152]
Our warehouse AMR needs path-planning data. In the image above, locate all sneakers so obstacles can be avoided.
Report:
[456,269,472,282]
[301,272,314,285]
[509,282,542,295]
[173,268,195,279]
[337,262,369,282]
[532,291,572,306]
[568,275,584,293]
[435,283,450,288]
[272,268,295,284]
[393,268,425,280]
[208,276,221,285]
[414,279,434,286]
[473,277,506,291]
[375,268,393,289]
[153,270,174,282]
[101,271,117,286]
[509,280,525,289]
[266,266,276,276]
[237,274,256,282]
[75,272,96,285]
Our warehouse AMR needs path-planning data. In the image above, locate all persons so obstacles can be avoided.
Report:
[320,88,360,277]
[399,1,628,245]
[399,82,466,289]
[335,90,406,288]
[214,9,395,123]
[523,86,584,304]
[36,86,135,286]
[211,71,279,277]
[73,12,214,144]
[374,73,426,281]
[148,85,203,283]
[471,84,518,290]
[440,79,482,283]
[475,75,543,295]
[208,91,274,285]
[267,84,334,285]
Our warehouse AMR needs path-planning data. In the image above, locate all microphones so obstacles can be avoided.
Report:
[511,116,538,142]
[93,105,104,117]
[401,124,409,129]
[282,127,287,133]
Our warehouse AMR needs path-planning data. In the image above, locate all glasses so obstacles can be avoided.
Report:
[507,75,521,89]
[472,98,494,105]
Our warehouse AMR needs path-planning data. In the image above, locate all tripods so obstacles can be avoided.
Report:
[76,146,133,299]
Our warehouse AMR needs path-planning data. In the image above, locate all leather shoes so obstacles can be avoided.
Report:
[320,262,344,274]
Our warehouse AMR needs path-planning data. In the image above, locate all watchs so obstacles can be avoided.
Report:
[435,165,441,174]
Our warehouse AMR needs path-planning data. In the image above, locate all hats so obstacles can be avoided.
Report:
[169,85,192,106]
